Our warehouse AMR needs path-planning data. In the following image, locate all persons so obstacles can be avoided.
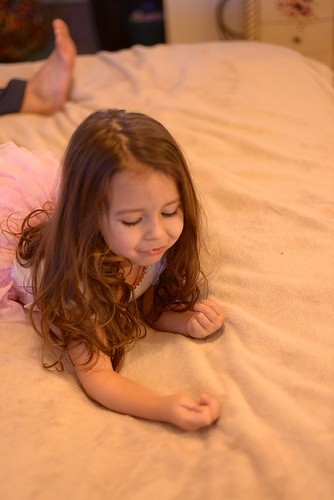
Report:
[0,18,77,115]
[0,108,225,431]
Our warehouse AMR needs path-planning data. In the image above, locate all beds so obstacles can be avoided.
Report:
[0,38,334,500]
